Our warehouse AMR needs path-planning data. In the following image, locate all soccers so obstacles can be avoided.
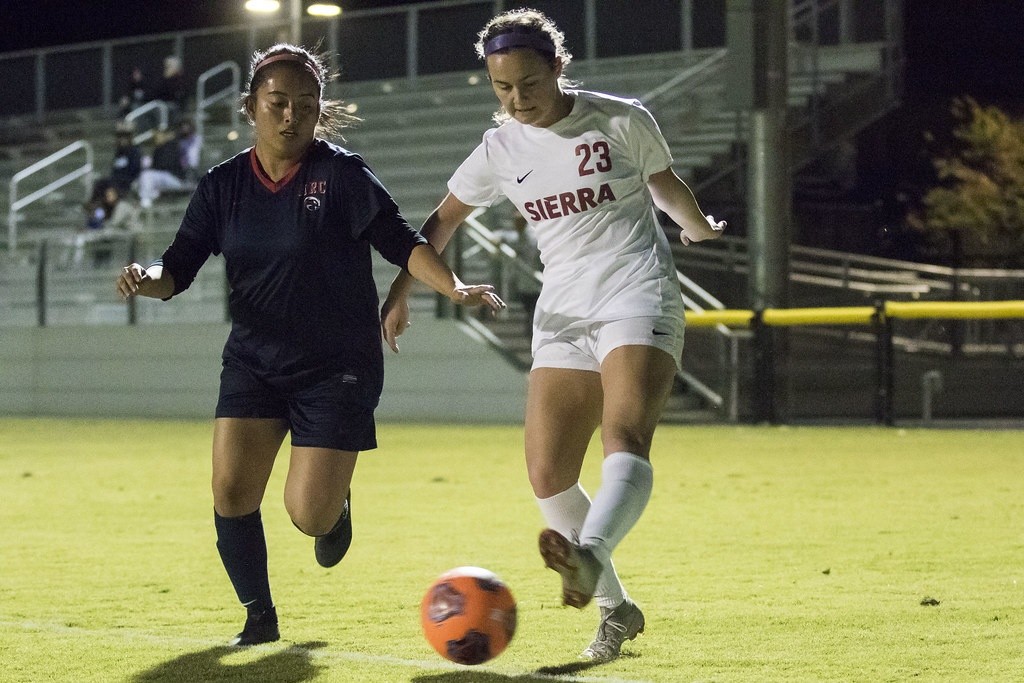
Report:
[418,564,521,667]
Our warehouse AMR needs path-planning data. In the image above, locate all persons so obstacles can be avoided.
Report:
[378,2,731,664]
[84,52,198,231]
[111,33,509,650]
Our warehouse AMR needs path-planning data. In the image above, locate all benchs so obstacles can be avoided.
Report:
[0,45,884,296]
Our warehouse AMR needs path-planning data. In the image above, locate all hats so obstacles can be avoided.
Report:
[115,121,134,132]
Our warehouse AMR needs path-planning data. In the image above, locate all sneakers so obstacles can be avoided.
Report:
[231,605,280,645]
[577,596,645,661]
[314,487,352,567]
[540,530,604,608]
